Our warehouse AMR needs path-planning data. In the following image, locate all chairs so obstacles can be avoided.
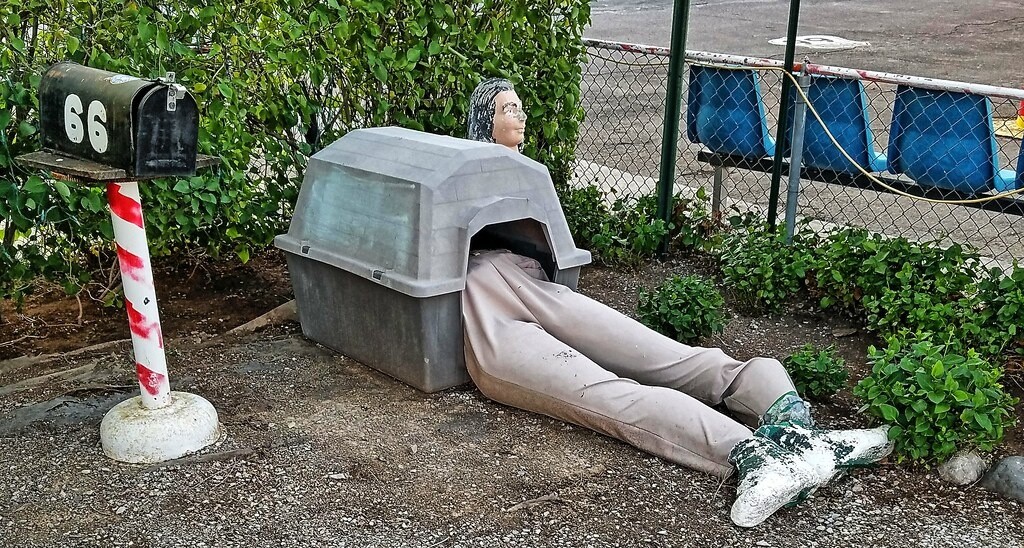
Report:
[687,63,1024,237]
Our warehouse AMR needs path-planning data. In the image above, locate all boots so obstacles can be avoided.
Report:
[757,391,895,466]
[727,437,820,527]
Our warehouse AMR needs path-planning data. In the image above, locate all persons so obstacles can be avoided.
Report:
[467,77,527,154]
[460,248,895,528]
